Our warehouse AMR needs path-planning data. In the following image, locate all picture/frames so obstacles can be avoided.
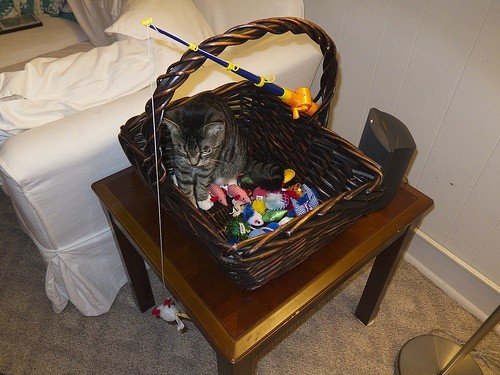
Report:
[0,11,44,34]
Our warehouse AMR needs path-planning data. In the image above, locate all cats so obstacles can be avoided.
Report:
[158,93,284,211]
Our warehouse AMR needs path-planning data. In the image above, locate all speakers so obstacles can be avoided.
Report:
[358,107,417,214]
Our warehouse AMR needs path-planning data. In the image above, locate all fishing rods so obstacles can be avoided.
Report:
[141,18,321,303]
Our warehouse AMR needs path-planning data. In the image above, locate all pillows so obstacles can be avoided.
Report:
[0,40,167,142]
[103,0,214,54]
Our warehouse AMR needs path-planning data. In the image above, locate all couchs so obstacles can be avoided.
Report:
[0,0,325,317]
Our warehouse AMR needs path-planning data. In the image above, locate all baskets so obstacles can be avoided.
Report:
[117,19,385,293]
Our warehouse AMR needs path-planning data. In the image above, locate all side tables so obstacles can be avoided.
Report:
[91,166,437,375]
